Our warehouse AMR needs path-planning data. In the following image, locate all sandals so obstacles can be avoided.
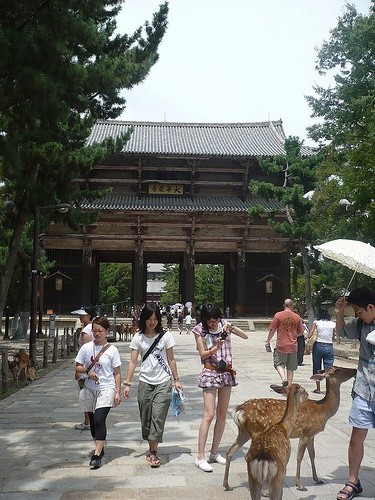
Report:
[337,478,363,500]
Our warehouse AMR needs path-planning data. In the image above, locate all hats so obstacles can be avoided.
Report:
[70,309,88,315]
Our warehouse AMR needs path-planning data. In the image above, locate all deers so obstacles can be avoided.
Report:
[27,367,39,381]
[223,365,358,491]
[245,381,309,500]
[116,322,137,342]
[13,352,32,388]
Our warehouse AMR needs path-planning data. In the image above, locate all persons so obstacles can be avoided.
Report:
[308,305,337,393]
[265,299,304,388]
[293,308,305,365]
[335,287,375,500]
[75,317,122,467]
[112,297,236,336]
[123,303,183,466]
[192,303,247,471]
[71,304,97,429]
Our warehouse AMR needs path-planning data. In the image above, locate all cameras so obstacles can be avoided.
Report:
[266,346,271,352]
[220,331,228,341]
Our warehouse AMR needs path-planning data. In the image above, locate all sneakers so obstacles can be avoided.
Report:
[146,450,161,467]
[90,446,104,466]
[195,452,227,471]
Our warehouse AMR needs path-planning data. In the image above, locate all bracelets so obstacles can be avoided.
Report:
[123,380,131,386]
[174,377,180,380]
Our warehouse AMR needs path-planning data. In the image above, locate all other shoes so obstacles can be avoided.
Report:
[75,422,90,430]
[314,389,321,393]
[282,379,289,387]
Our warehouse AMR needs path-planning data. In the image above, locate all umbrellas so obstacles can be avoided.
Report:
[313,239,375,312]
